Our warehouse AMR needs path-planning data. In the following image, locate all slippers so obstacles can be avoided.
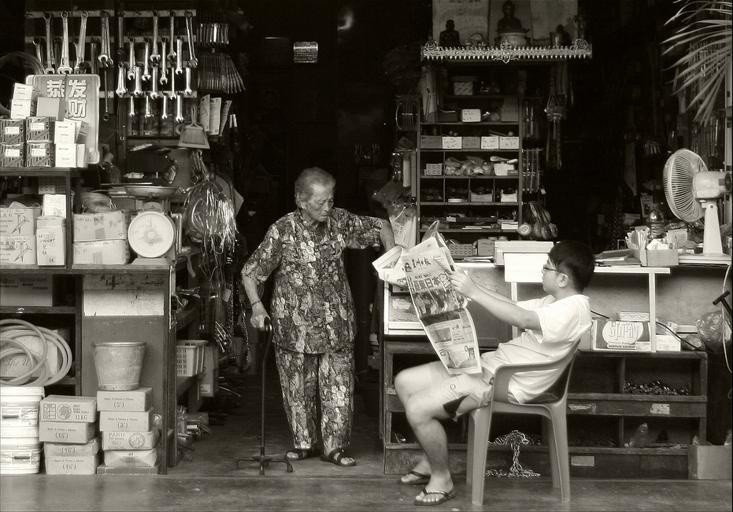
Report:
[414,487,458,505]
[400,471,430,485]
[285,449,313,461]
[320,448,356,467]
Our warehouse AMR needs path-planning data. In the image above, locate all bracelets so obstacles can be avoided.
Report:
[250,298,264,310]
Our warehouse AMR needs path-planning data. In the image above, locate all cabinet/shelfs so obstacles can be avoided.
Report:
[0,163,233,476]
[415,95,536,245]
[384,241,733,477]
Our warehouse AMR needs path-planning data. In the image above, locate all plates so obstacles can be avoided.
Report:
[127,212,175,257]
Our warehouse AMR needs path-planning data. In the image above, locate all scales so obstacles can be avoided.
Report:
[126,185,179,267]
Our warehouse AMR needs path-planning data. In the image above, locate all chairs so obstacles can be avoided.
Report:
[465,341,580,508]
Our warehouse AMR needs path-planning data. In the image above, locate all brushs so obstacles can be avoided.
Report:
[182,104,205,144]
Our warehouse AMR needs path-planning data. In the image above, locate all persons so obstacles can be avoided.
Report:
[439,19,460,49]
[240,166,399,465]
[393,241,595,506]
[497,1,523,39]
[413,291,448,316]
